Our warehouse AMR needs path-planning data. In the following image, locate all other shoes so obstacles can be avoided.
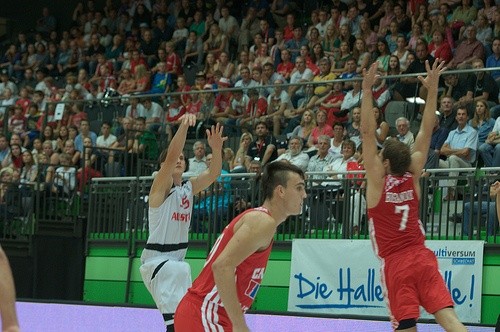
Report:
[443,192,454,201]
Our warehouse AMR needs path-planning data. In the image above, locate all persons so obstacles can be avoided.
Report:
[358,60,469,332]
[0,0,500,332]
